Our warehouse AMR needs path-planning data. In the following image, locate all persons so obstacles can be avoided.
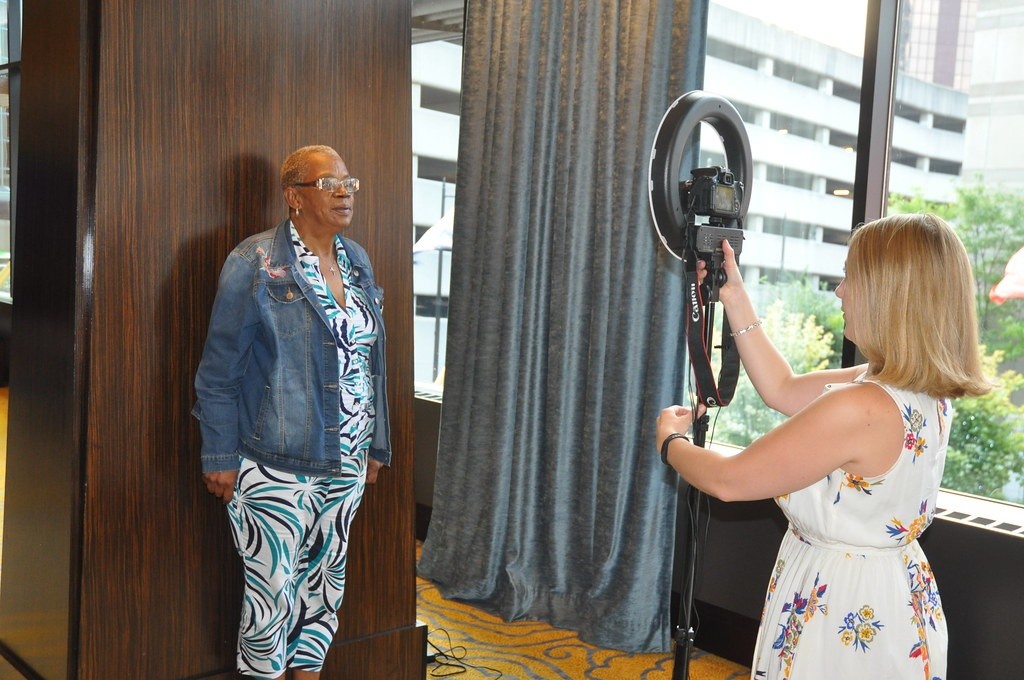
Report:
[191,143,390,680]
[655,214,989,680]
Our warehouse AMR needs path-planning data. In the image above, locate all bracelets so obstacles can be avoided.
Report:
[661,433,690,466]
[730,320,762,337]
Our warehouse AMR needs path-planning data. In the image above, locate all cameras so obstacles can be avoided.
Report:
[678,166,743,226]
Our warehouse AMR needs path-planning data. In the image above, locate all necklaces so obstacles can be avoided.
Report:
[320,260,337,276]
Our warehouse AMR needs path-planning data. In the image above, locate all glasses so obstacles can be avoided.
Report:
[294,176,360,193]
